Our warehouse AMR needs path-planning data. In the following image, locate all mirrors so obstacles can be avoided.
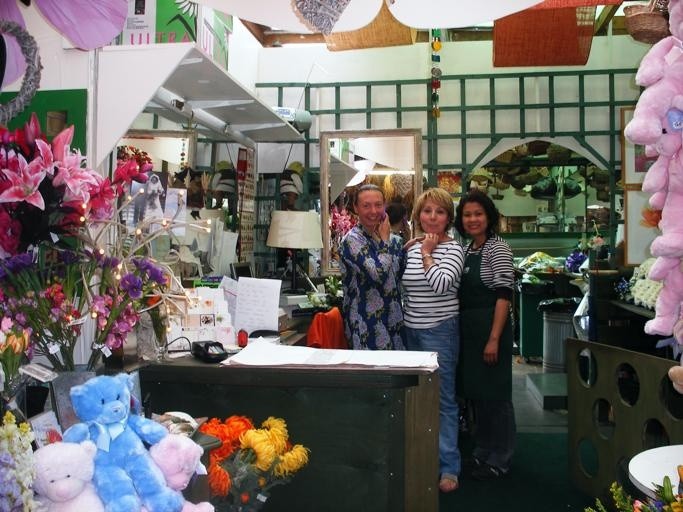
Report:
[116,130,198,182]
[319,127,424,279]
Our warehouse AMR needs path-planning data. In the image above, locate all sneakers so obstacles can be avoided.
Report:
[440,473,460,493]
[478,465,514,481]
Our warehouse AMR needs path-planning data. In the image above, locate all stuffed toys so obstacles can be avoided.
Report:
[146,433,217,512]
[61,372,186,512]
[620,1,682,397]
[25,439,105,511]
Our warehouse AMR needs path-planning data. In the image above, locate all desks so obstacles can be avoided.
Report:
[139,349,441,511]
[627,444,683,501]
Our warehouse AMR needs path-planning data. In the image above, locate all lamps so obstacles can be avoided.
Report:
[265,210,324,295]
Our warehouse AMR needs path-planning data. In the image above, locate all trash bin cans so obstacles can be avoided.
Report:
[518,278,560,364]
[542,297,582,373]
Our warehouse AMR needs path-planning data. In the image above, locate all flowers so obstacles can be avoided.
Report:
[198,415,312,510]
[117,146,153,167]
[583,466,683,512]
[329,209,352,244]
[1,408,35,511]
[1,113,175,414]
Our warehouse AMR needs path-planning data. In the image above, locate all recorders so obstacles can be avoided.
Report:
[271,108,311,132]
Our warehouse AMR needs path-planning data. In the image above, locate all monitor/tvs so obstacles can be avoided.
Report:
[229,262,255,282]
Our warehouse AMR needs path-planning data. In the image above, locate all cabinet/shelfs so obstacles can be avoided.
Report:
[98,38,310,338]
[327,153,359,205]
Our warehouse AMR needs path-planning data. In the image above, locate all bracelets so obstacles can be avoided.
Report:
[422,253,432,259]
[423,259,433,266]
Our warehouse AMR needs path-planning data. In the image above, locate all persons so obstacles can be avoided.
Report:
[401,188,465,492]
[335,183,426,350]
[455,191,517,477]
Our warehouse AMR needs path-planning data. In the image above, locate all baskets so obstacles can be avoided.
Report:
[624,0,669,44]
[471,140,610,201]
